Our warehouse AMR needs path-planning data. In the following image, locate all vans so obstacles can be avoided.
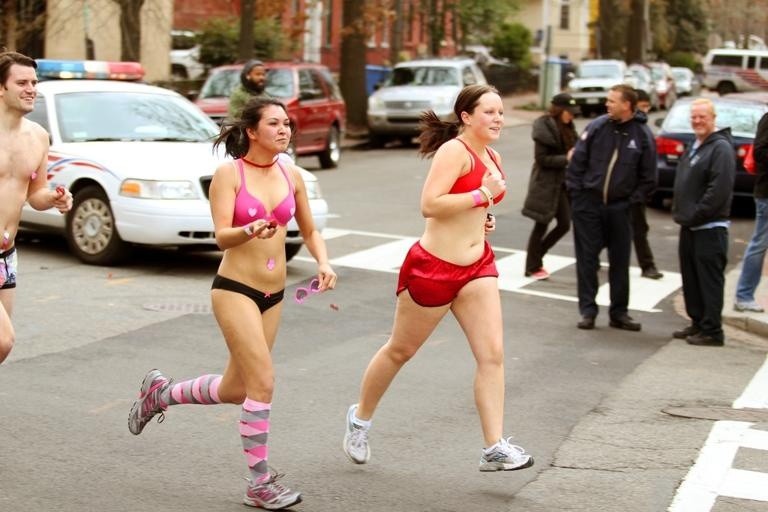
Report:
[700,46,768,95]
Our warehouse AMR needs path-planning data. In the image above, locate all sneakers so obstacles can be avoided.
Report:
[641,267,663,279]
[478,435,534,473]
[673,325,700,340]
[524,268,550,280]
[242,465,304,509]
[686,331,727,347]
[342,404,371,466]
[733,300,765,314]
[128,368,173,436]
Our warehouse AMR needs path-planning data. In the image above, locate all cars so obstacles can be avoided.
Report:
[626,64,659,110]
[647,61,677,111]
[672,68,700,97]
[16,59,327,267]
[646,95,768,219]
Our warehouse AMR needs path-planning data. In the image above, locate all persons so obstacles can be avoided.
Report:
[127,99,340,510]
[521,92,581,279]
[342,84,535,469]
[565,85,657,330]
[0,51,63,364]
[671,97,735,347]
[227,59,271,124]
[630,89,663,280]
[732,103,768,312]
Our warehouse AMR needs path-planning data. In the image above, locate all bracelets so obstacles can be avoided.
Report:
[479,185,494,209]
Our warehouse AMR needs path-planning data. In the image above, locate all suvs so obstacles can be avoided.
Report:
[564,59,628,119]
[192,62,347,168]
[170,30,207,81]
[368,58,488,150]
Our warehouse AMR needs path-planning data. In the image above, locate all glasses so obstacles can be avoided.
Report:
[294,279,321,302]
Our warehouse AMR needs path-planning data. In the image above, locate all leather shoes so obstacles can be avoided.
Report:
[608,312,642,331]
[577,313,596,329]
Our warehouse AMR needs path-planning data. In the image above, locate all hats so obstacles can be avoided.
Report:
[550,92,582,115]
[241,59,264,76]
